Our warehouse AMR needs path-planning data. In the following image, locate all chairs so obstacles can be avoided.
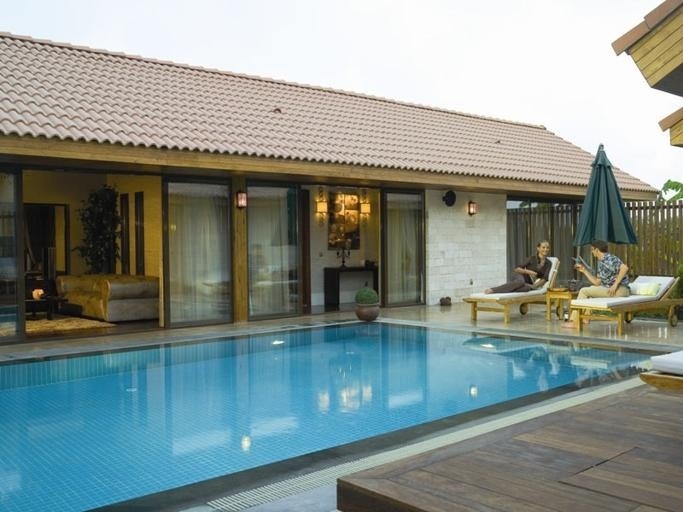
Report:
[462,257,561,325]
[568,274,682,336]
[640,350,683,391]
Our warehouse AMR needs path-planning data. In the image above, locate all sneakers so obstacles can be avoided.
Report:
[441,297,451,306]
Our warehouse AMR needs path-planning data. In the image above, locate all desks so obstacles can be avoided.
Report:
[324,265,378,311]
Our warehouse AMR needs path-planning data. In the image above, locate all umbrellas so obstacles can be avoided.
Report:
[573,143,637,278]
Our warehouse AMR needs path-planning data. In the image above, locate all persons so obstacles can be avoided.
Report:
[560,238,631,330]
[481,238,552,295]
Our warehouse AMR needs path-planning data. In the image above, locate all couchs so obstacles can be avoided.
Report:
[55,273,159,323]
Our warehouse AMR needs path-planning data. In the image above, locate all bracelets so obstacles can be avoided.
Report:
[612,284,617,289]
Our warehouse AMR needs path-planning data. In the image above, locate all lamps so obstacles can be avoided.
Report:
[336,233,352,267]
[316,185,328,225]
[236,190,248,209]
[468,200,477,215]
[360,189,371,227]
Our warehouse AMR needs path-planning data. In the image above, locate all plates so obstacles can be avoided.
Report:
[547,288,569,292]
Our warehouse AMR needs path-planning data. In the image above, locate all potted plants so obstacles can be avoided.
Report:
[355,288,380,320]
